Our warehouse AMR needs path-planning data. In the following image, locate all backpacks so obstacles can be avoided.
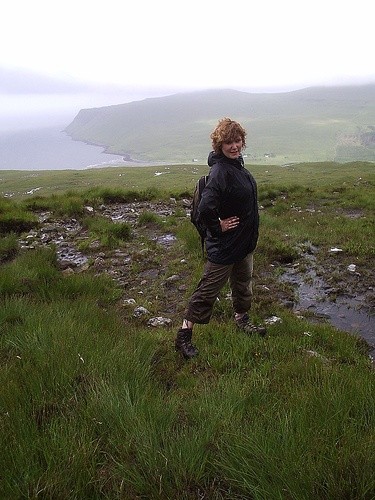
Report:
[190,176,209,257]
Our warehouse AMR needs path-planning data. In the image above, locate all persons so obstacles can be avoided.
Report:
[175,116,266,359]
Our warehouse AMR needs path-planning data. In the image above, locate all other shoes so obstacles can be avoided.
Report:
[236,316,266,337]
[176,327,199,359]
